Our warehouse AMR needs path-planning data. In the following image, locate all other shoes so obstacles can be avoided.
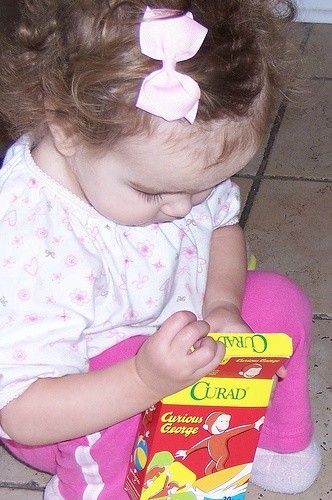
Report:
[252,439,321,494]
[43,475,62,499]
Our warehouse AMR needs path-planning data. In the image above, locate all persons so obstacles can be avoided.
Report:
[0,0,322,499]
